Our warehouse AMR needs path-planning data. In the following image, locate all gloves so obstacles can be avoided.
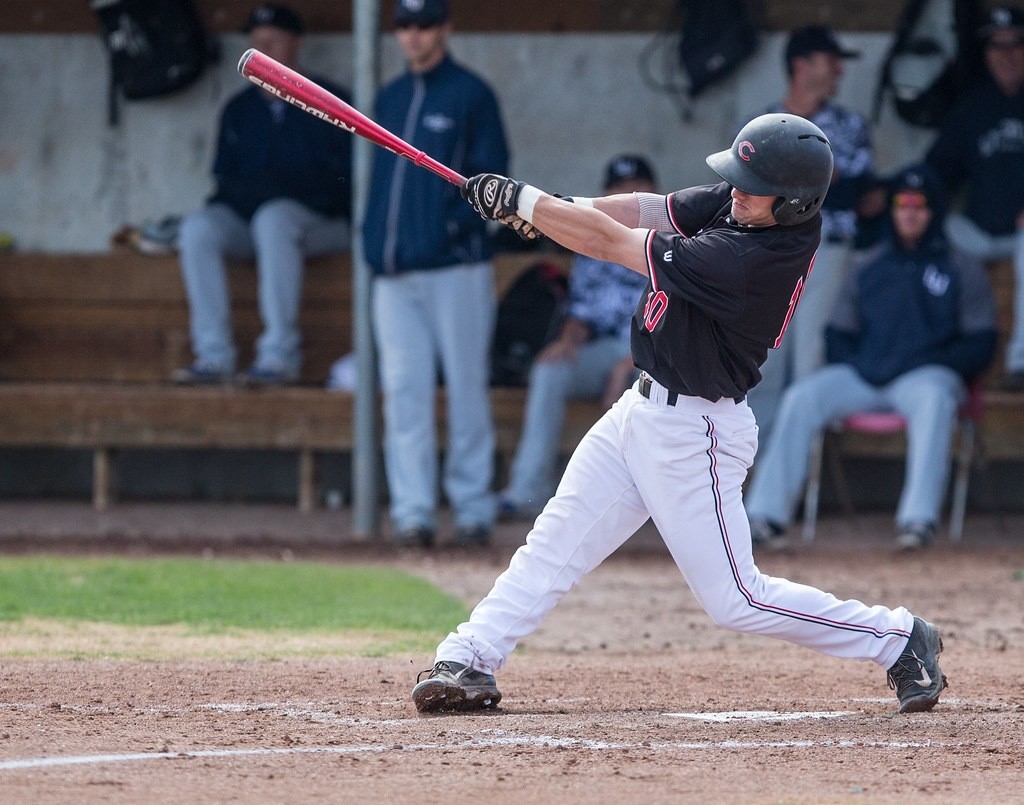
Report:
[497,189,573,243]
[461,174,526,221]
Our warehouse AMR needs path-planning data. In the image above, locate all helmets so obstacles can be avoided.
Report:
[706,112,834,225]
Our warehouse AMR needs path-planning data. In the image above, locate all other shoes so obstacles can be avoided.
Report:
[750,522,780,543]
[450,525,489,544]
[398,527,430,546]
[174,368,219,386]
[899,525,928,544]
[235,366,291,387]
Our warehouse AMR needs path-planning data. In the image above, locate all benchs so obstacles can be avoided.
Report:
[1,247,1023,514]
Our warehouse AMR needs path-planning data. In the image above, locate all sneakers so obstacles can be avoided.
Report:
[412,661,501,709]
[886,617,944,711]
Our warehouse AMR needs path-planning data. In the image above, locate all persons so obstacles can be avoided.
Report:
[412,112,949,714]
[361,0,510,548]
[733,27,879,461]
[490,155,657,521]
[743,164,998,551]
[920,7,1024,389]
[98,0,224,216]
[170,3,355,383]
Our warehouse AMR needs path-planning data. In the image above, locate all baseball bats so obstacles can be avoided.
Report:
[235,46,517,222]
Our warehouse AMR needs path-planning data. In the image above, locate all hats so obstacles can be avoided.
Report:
[894,173,927,194]
[243,6,304,35]
[604,155,652,190]
[397,0,444,23]
[786,25,859,58]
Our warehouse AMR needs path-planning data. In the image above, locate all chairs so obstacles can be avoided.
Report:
[801,374,993,545]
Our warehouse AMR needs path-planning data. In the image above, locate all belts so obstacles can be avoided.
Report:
[637,373,745,405]
